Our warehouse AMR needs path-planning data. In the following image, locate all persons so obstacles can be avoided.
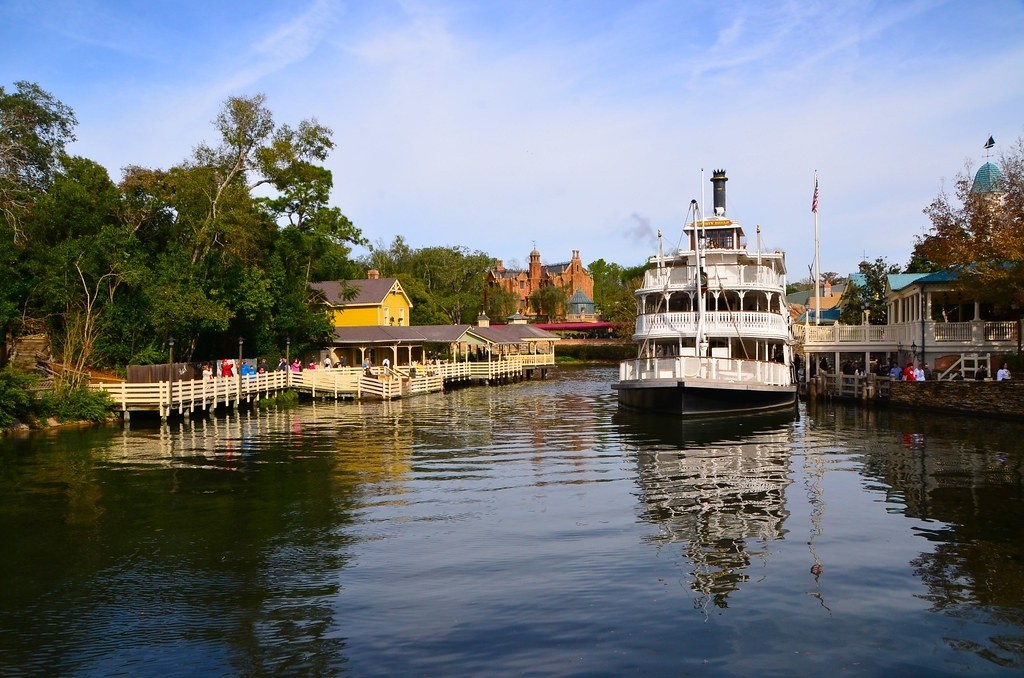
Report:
[790,353,1012,383]
[340,355,346,366]
[382,357,390,373]
[292,358,301,372]
[363,358,370,368]
[277,358,290,371]
[202,358,269,380]
[324,354,331,367]
[309,358,316,369]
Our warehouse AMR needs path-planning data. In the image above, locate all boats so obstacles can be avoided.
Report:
[610,165,798,417]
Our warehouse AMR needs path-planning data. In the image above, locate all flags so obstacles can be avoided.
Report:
[812,181,819,212]
